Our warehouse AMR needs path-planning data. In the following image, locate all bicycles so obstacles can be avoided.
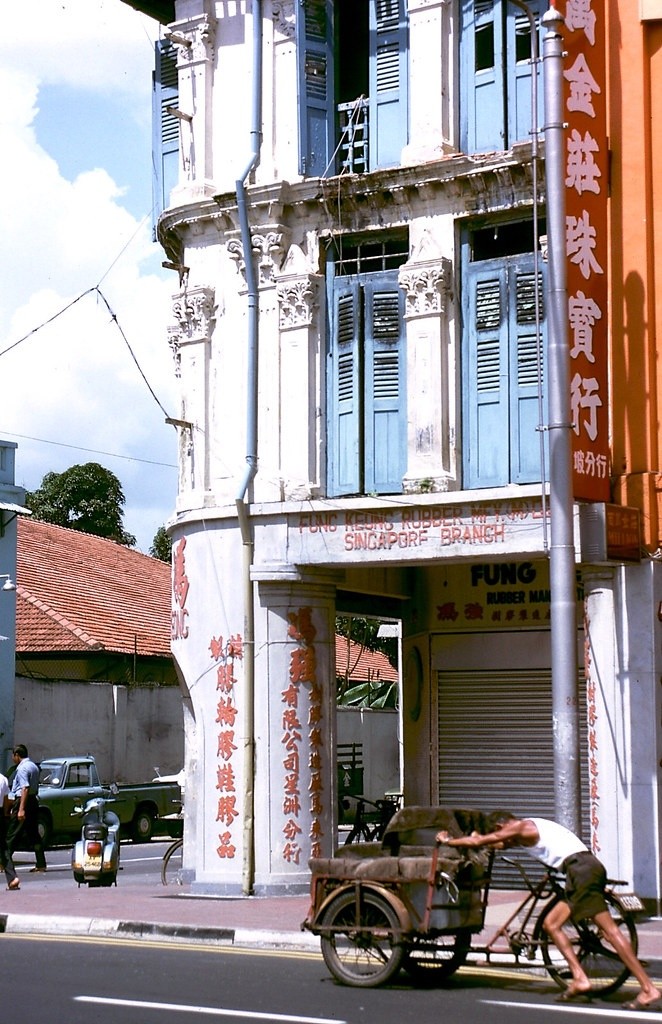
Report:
[345,792,403,848]
[160,800,184,887]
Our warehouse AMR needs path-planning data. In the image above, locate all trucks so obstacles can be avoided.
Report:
[2,755,184,851]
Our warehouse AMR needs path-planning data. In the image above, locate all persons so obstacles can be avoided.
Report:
[0,744,47,890]
[436,810,660,1008]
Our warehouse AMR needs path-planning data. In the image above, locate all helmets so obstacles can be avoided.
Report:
[81,812,102,827]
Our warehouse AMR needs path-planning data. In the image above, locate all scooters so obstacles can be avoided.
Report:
[72,780,122,889]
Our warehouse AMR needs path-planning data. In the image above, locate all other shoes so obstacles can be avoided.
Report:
[29,867,46,873]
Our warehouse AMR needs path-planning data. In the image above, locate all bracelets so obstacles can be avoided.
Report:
[444,837,449,843]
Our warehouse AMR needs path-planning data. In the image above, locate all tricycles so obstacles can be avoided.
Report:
[299,806,647,998]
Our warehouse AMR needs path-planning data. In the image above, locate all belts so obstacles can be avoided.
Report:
[562,850,592,867]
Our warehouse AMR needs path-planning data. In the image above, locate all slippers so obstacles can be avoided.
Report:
[553,988,594,1004]
[5,881,21,891]
[620,995,662,1013]
